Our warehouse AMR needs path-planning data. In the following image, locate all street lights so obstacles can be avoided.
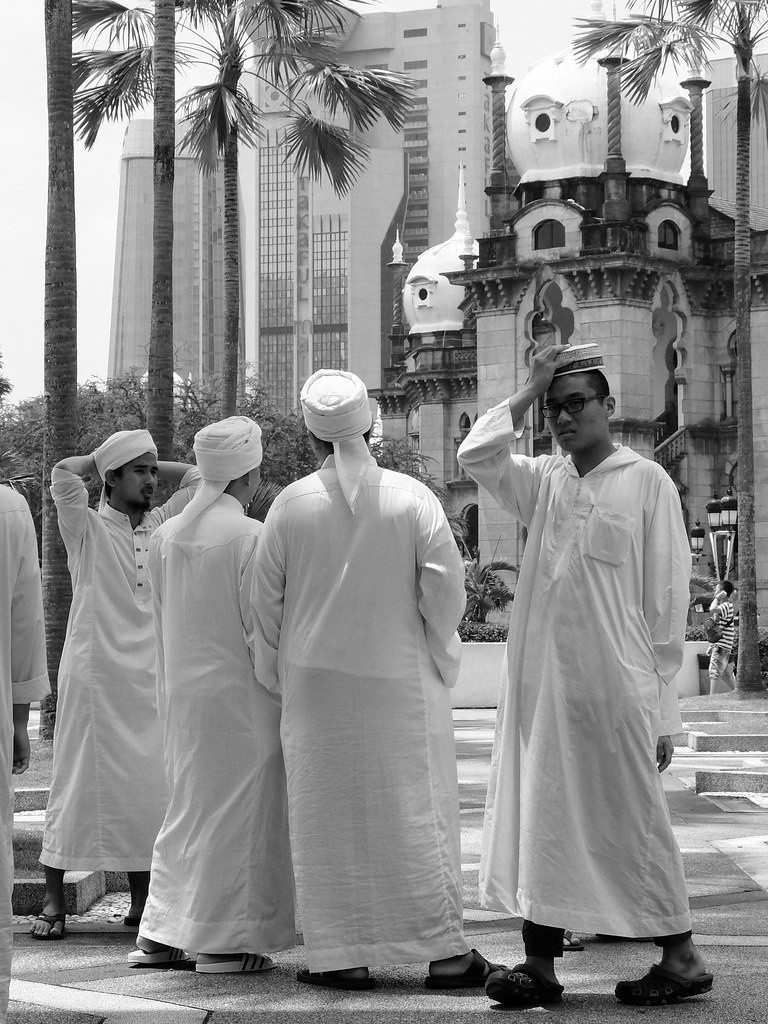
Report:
[690,518,706,569]
[705,485,738,582]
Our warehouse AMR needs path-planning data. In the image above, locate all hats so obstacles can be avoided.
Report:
[94,430,159,514]
[299,369,373,515]
[553,343,605,377]
[161,415,263,557]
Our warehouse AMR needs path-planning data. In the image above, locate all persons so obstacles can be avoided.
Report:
[28,429,199,939]
[249,368,513,988]
[457,343,713,1005]
[128,414,295,973]
[707,581,735,695]
[0,483,52,1024]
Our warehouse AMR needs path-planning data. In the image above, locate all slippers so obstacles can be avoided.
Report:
[32,912,65,939]
[562,930,584,951]
[614,963,714,1005]
[424,948,510,990]
[128,946,188,963]
[296,969,376,990]
[196,953,278,973]
[123,915,141,926]
[485,963,564,1007]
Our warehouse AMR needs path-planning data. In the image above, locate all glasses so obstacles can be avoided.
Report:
[541,395,610,418]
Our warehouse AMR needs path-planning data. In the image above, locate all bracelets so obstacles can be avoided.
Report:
[715,597,720,601]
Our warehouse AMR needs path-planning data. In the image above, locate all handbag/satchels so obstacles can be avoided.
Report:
[704,606,734,643]
